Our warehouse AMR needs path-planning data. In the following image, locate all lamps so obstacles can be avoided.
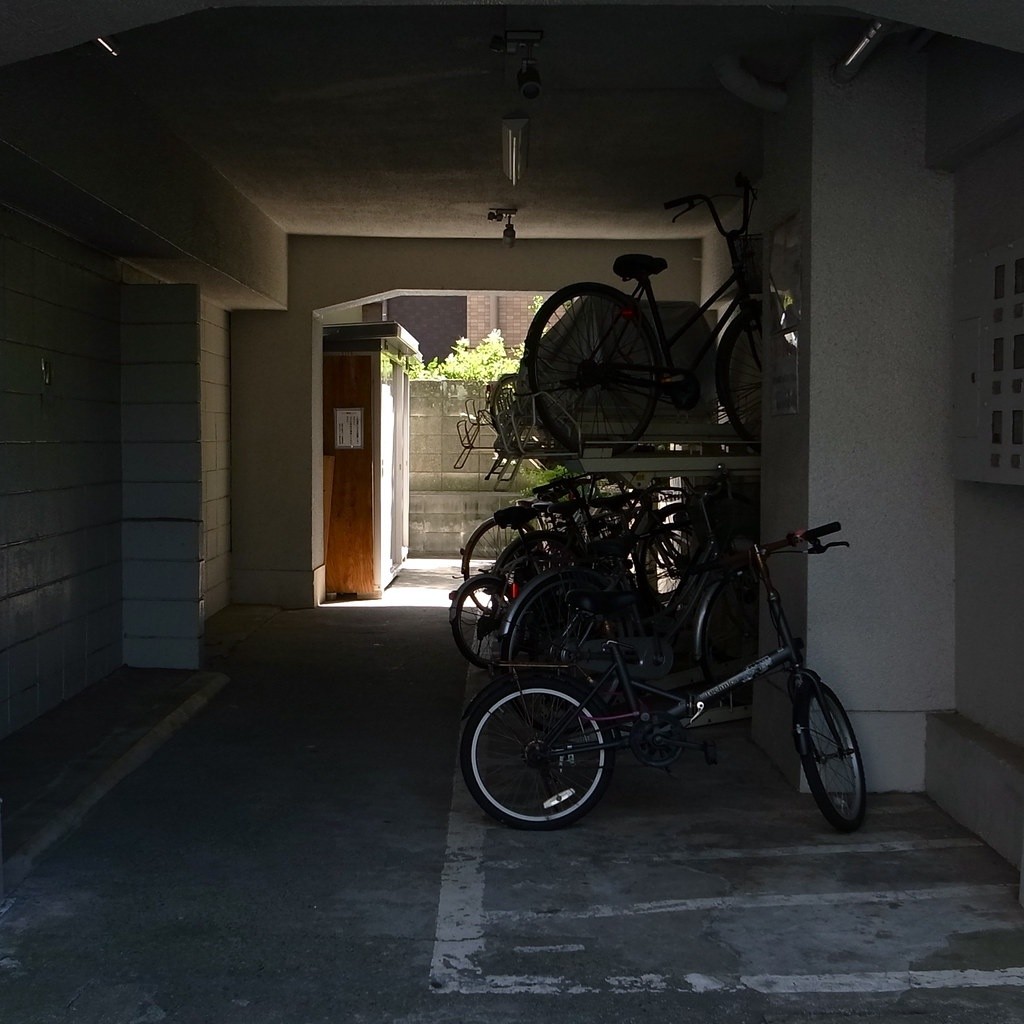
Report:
[489,209,517,248]
[488,30,545,101]
[502,113,529,186]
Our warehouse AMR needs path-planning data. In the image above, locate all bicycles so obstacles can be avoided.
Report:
[460,520,867,835]
[448,171,765,736]
